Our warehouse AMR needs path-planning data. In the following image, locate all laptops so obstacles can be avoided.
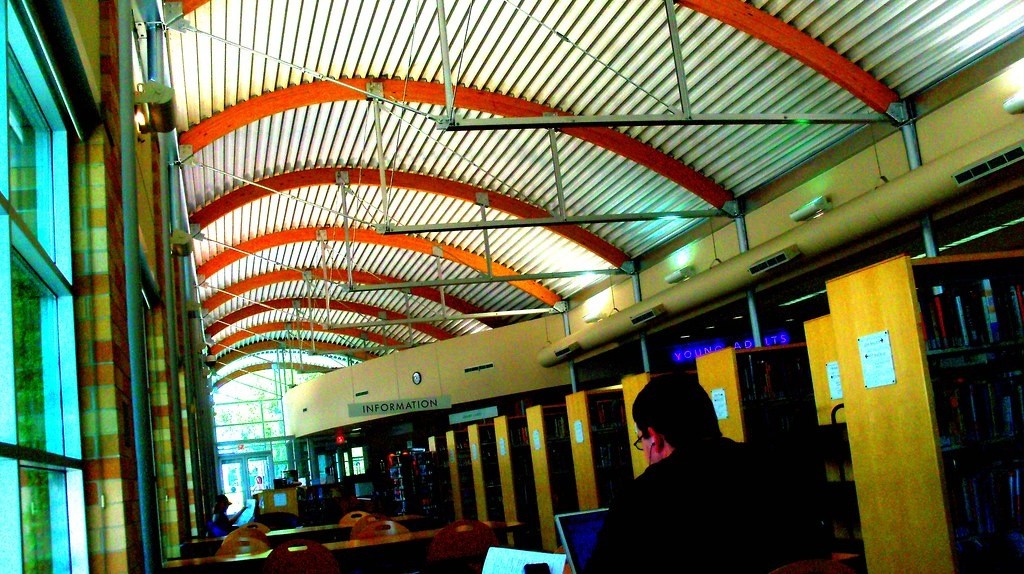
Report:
[554,508,609,574]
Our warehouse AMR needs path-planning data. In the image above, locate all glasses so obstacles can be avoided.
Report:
[634,435,644,450]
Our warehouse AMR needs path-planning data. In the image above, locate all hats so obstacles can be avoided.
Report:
[215,494,232,505]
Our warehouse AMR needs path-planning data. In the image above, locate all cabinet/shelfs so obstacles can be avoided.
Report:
[494,415,541,546]
[428,436,448,469]
[826,250,1024,574]
[696,341,808,443]
[468,423,505,521]
[446,430,477,521]
[622,370,697,481]
[565,388,634,511]
[525,403,579,552]
[804,313,863,540]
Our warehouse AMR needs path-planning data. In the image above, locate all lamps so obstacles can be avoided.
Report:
[134,79,176,133]
[582,310,605,324]
[790,196,832,222]
[170,229,193,256]
[664,265,692,284]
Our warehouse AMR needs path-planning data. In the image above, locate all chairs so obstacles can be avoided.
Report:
[216,522,270,555]
[265,539,340,574]
[428,521,500,574]
[339,511,410,540]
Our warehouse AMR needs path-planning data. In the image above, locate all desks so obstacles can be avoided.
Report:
[163,521,522,567]
[186,515,423,544]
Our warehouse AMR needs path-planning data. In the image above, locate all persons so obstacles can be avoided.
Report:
[583,374,834,574]
[253,476,264,506]
[212,494,248,534]
